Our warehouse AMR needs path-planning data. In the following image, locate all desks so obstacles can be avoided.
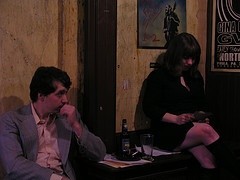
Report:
[85,131,199,180]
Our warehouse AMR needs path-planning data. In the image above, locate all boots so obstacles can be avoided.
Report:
[200,167,220,180]
[205,136,240,177]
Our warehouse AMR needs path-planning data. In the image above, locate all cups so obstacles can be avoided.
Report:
[140,134,153,160]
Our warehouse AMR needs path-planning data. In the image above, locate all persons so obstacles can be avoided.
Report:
[141,32,240,180]
[0,66,106,180]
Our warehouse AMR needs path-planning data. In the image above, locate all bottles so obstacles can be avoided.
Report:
[119,119,132,160]
